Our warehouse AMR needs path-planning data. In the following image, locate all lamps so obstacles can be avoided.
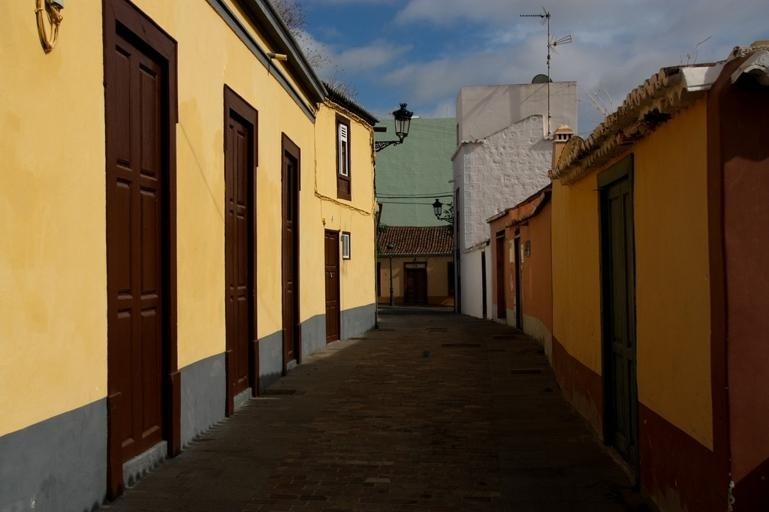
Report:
[431,198,455,224]
[371,100,414,153]
